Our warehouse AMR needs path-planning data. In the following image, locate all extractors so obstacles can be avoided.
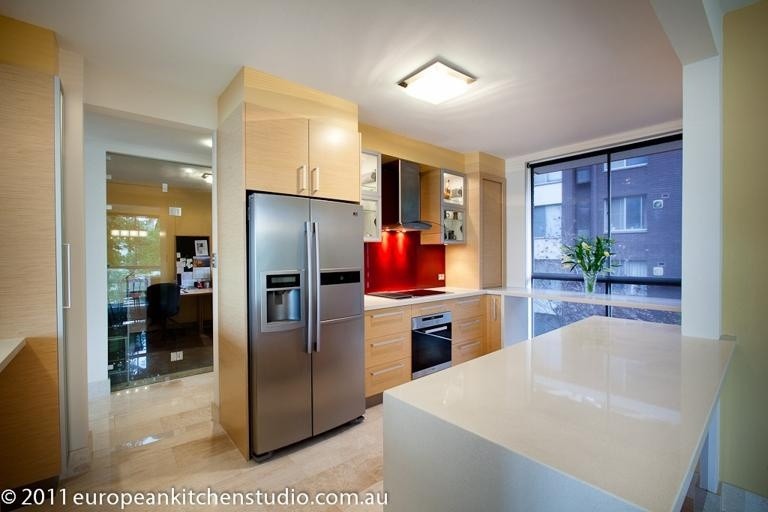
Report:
[381,158,432,234]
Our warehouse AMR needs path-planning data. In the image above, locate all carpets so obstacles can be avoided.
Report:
[148,319,212,354]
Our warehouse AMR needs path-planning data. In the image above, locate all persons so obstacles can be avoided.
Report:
[446,179,451,200]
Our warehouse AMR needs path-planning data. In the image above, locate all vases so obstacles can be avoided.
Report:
[582,270,596,294]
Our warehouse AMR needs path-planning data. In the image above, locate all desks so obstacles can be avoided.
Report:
[179,286,212,340]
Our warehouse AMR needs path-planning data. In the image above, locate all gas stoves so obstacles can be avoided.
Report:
[367,289,455,300]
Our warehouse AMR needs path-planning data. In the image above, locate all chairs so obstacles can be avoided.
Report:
[145,281,185,341]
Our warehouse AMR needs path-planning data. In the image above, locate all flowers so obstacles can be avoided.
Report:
[556,233,625,293]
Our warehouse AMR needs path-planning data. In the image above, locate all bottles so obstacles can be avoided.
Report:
[444,180,451,199]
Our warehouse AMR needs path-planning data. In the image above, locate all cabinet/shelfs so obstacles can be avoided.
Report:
[450,296,501,366]
[445,148,508,292]
[364,308,411,397]
[217,65,362,204]
[418,165,466,246]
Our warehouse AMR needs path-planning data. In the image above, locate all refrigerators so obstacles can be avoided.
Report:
[249,192,365,461]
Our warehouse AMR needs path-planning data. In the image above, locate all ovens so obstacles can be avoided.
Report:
[410,310,452,380]
